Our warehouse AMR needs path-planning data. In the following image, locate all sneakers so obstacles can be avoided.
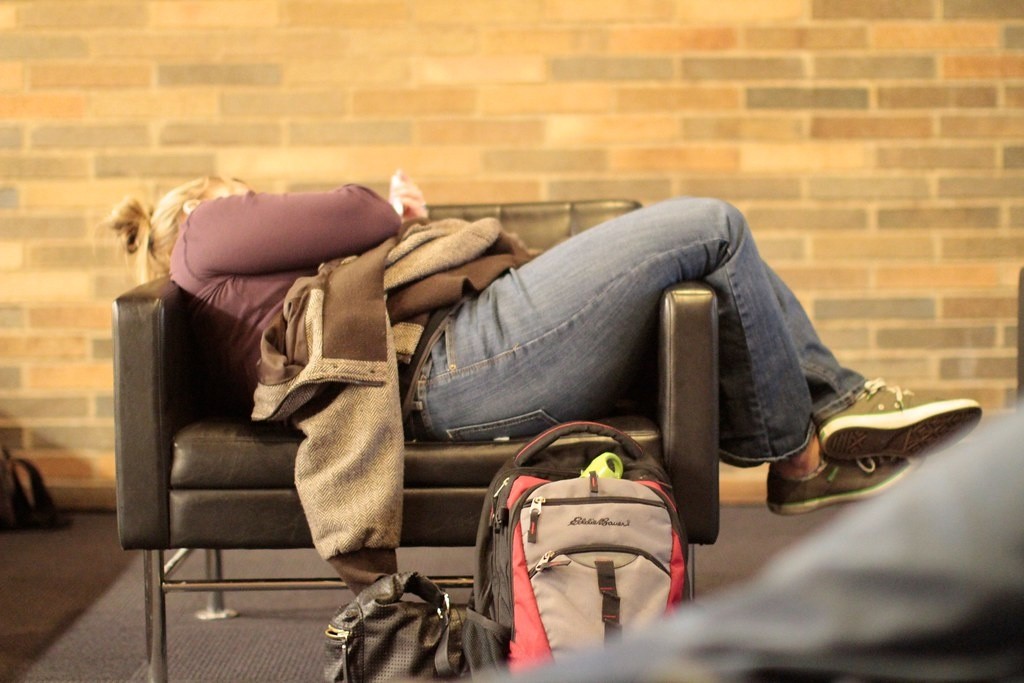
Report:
[766,381,982,514]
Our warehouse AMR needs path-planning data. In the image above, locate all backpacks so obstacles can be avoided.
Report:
[462,421,693,680]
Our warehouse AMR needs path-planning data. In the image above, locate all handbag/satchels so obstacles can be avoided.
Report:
[322,571,506,682]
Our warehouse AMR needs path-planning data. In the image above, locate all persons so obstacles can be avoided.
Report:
[108,175,982,516]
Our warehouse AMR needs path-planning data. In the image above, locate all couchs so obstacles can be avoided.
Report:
[106,196,724,683]
